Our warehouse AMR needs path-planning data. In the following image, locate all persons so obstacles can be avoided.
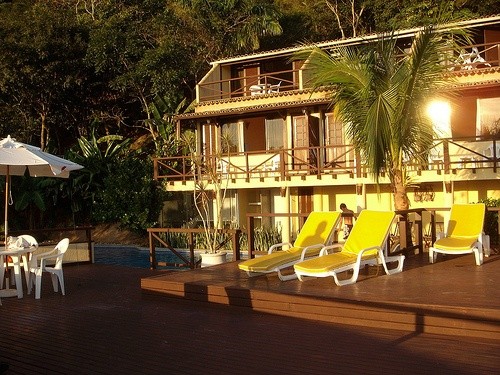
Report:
[337,203,357,242]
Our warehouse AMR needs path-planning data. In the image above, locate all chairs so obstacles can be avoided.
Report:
[293,209,405,286]
[428,153,444,170]
[238,212,341,281]
[262,161,279,176]
[28,238,70,299]
[427,203,490,266]
[270,81,282,93]
[250,85,262,96]
[483,143,500,168]
[0,234,38,295]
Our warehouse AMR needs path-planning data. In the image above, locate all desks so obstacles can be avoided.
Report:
[0,246,36,297]
[258,84,271,94]
[459,157,476,169]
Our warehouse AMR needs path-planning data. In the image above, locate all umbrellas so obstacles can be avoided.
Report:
[0,135,84,278]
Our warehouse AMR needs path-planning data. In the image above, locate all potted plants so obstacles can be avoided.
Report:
[178,130,237,268]
